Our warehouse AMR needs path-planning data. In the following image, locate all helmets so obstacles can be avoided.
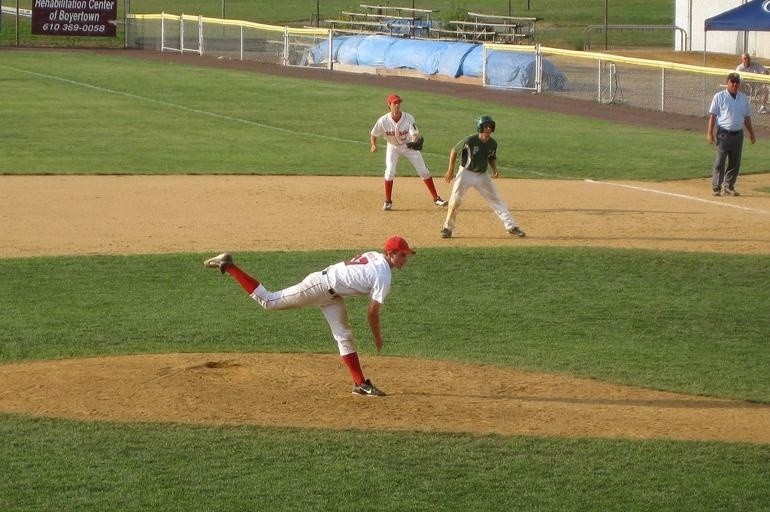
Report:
[475,115,496,133]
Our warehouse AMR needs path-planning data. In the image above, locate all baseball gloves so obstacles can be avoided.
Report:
[406,136,424,150]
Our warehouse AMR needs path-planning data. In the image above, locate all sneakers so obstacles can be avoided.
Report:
[510,227,525,237]
[383,202,392,210]
[723,184,740,196]
[203,252,233,274]
[712,184,722,196]
[352,378,386,397]
[440,227,453,238]
[433,196,448,207]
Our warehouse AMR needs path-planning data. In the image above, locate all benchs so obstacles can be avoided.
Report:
[265,4,545,58]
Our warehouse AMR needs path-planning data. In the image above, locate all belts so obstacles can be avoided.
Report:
[721,129,743,135]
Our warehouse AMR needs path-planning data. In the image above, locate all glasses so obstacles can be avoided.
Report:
[729,79,739,84]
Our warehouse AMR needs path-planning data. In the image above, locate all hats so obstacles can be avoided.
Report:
[726,72,740,83]
[387,94,401,106]
[383,235,416,256]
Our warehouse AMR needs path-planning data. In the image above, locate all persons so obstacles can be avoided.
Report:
[440,115,526,237]
[370,94,448,211]
[707,73,756,196]
[736,53,769,114]
[204,235,416,397]
